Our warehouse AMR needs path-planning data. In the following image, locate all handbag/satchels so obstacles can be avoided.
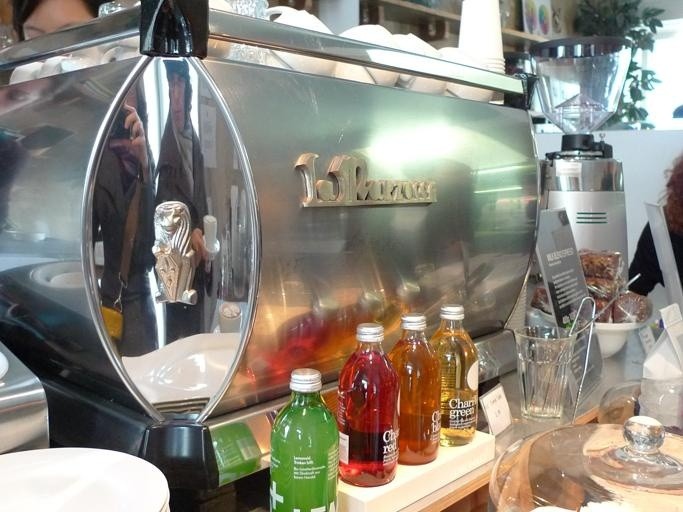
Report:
[101,306,124,340]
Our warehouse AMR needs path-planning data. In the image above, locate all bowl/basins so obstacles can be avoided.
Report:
[592,320,642,363]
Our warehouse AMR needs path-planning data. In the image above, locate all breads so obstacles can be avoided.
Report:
[531,250,652,324]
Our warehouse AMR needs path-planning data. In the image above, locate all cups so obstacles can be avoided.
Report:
[268,4,492,103]
[459,0,506,109]
[217,302,243,333]
[512,323,576,424]
[9,0,268,84]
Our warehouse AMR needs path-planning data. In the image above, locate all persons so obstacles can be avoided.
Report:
[89,76,160,358]
[0,69,79,241]
[12,0,115,41]
[409,160,479,279]
[154,58,222,348]
[627,153,682,296]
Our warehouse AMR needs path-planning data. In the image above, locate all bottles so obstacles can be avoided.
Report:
[335,317,398,491]
[426,302,481,450]
[385,315,442,467]
[264,367,340,512]
[387,277,420,331]
[244,296,341,385]
[308,288,387,369]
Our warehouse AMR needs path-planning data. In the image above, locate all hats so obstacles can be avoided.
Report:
[163,58,188,76]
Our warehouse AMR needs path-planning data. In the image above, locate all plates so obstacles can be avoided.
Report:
[118,332,245,411]
[1,446,170,512]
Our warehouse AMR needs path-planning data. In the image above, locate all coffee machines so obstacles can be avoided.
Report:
[530,34,638,299]
